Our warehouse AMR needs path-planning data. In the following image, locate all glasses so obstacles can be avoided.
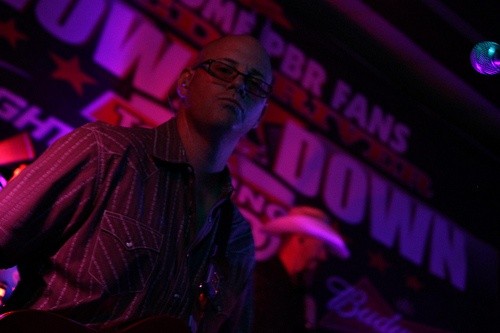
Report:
[193,59,272,100]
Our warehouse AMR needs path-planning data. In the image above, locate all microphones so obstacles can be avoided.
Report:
[470,41,500,75]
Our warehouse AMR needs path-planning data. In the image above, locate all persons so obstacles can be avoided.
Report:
[0,35,274,333]
[254,206,349,333]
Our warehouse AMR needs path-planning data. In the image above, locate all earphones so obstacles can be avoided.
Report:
[182,80,188,89]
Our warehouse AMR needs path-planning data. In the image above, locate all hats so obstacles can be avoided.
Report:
[262,206,349,259]
